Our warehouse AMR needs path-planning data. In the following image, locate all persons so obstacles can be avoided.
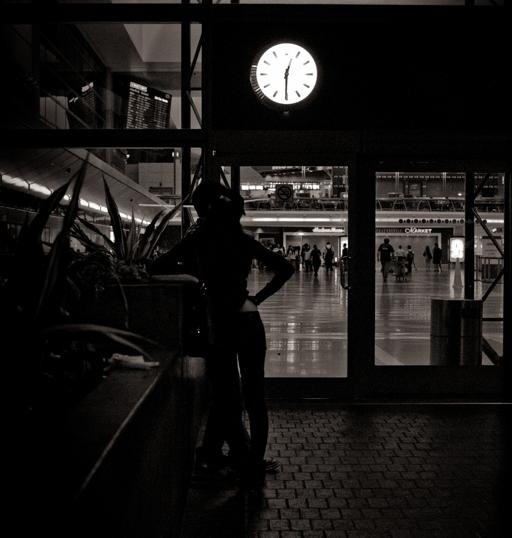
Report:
[148,180,297,508]
[266,193,344,210]
[423,243,441,272]
[342,244,348,271]
[377,238,414,282]
[252,239,334,276]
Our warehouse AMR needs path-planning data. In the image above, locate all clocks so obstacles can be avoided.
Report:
[249,38,324,111]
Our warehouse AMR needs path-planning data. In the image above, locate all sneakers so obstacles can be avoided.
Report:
[193,447,280,513]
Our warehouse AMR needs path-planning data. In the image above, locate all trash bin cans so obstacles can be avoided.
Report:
[430,299,483,367]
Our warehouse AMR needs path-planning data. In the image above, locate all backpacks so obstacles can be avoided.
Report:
[407,251,414,261]
[325,245,333,261]
